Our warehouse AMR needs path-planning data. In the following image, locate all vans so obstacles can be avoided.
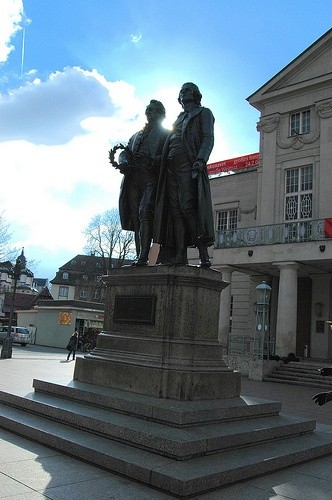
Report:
[0,325,31,347]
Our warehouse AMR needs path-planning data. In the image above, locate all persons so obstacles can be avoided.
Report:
[66,331,77,360]
[312,368,332,406]
[119,100,171,266]
[154,82,215,268]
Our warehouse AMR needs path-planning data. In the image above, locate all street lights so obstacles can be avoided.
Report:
[1,258,22,359]
[251,281,272,360]
